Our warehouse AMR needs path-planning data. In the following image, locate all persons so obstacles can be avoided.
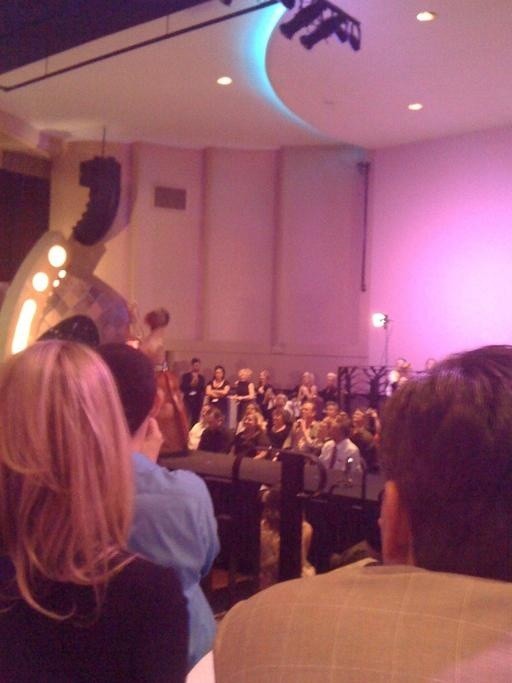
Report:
[214,343,511,681]
[0,344,229,682]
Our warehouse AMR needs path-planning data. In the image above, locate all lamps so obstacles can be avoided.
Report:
[1,231,76,358]
[273,0,362,54]
[371,312,393,370]
